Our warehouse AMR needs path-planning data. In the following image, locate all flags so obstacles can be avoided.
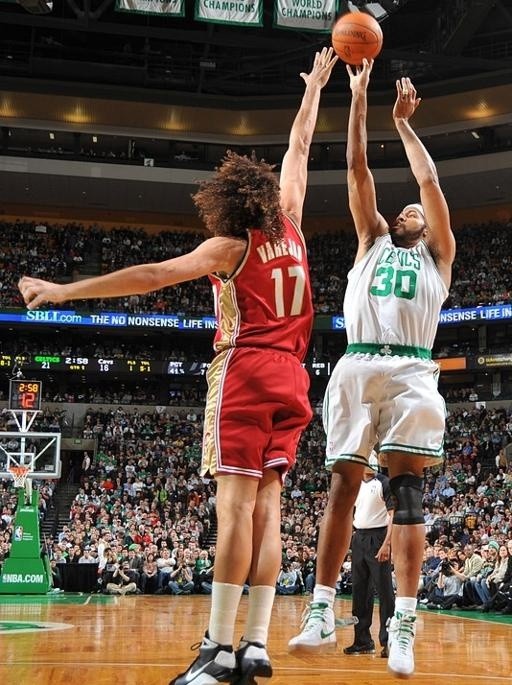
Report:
[193,0,264,29]
[272,0,340,34]
[115,0,187,19]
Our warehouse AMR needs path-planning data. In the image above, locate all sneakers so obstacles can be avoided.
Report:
[342,640,376,655]
[379,644,391,658]
[169,630,237,685]
[384,608,417,677]
[230,634,274,685]
[288,601,338,648]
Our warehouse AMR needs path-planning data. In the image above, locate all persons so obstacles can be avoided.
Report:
[287,55,457,674]
[342,445,398,659]
[0,132,510,614]
[14,43,339,683]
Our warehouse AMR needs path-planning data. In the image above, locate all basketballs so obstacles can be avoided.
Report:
[332,11,382,65]
